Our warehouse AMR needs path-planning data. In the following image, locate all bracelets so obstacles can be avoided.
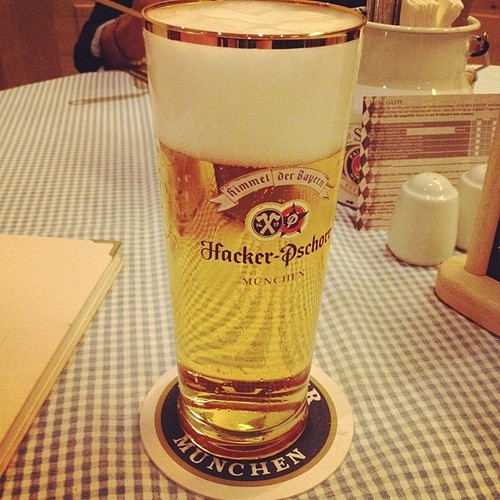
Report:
[108,14,146,67]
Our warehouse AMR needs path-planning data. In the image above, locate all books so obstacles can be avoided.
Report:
[1,231,124,480]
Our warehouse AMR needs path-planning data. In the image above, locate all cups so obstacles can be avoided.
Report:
[141,2,367,460]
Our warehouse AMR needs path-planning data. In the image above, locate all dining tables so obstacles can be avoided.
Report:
[1,68,498,500]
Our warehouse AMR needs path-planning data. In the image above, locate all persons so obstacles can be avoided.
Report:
[72,0,368,76]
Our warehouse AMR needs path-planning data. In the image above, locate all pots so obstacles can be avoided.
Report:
[353,5,492,89]
